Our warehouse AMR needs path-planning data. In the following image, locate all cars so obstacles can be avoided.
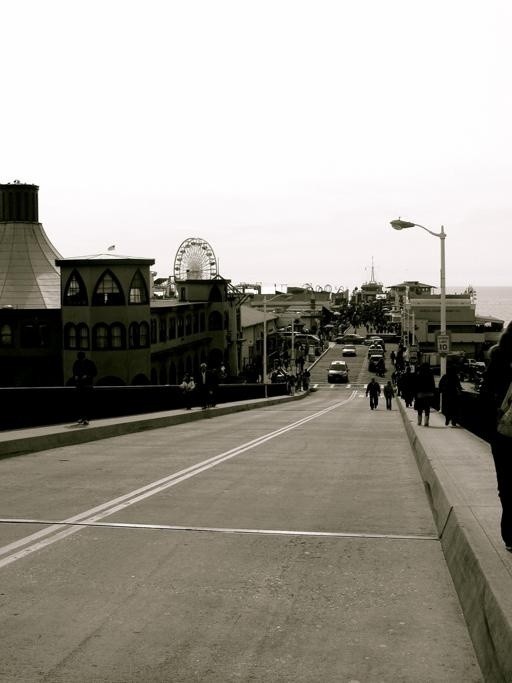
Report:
[364,335,385,371]
[342,343,357,357]
[327,360,351,383]
[335,335,365,344]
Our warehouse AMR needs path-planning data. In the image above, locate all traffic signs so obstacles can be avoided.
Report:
[436,334,450,354]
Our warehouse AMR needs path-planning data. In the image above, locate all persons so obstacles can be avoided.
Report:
[182,373,196,409]
[72,350,97,426]
[391,342,414,410]
[437,367,461,426]
[366,379,381,410]
[384,381,395,410]
[479,319,512,551]
[414,362,435,427]
[303,369,310,389]
[285,373,303,397]
[198,363,214,409]
[376,358,386,377]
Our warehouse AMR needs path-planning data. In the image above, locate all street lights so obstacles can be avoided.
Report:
[291,309,301,372]
[389,217,448,414]
[263,292,294,398]
[400,302,417,349]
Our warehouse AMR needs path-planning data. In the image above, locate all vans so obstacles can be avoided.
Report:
[294,334,320,345]
